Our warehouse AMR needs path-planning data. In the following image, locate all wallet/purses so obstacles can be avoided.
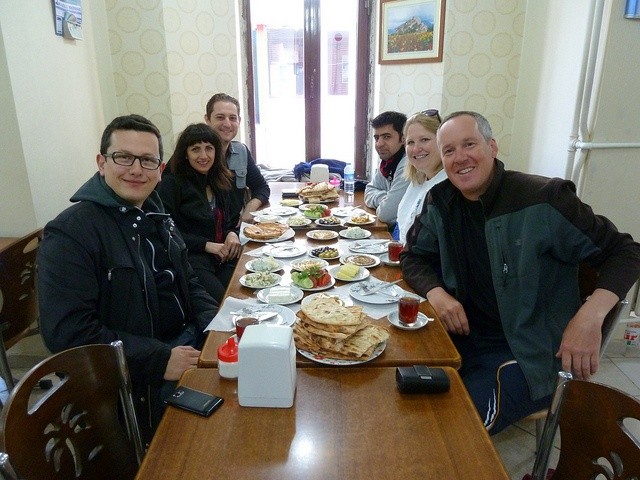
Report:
[395,365,450,394]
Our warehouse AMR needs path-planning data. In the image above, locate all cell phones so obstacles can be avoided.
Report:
[162,385,223,416]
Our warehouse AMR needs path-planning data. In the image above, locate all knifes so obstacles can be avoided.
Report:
[228,312,277,332]
[361,278,404,296]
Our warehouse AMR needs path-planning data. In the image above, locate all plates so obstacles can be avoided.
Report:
[331,265,370,282]
[281,216,312,226]
[339,228,371,239]
[348,281,405,304]
[243,222,295,242]
[343,216,375,225]
[301,210,334,219]
[315,217,346,227]
[263,241,307,258]
[306,230,339,240]
[387,310,429,330]
[298,196,339,203]
[232,304,296,327]
[301,291,353,310]
[245,257,284,272]
[238,272,283,288]
[297,338,387,367]
[263,207,297,216]
[347,239,393,253]
[257,286,304,305]
[299,204,328,211]
[283,199,303,207]
[380,253,401,266]
[289,274,336,291]
[290,258,329,271]
[332,206,366,217]
[308,246,344,259]
[339,253,381,267]
[253,215,283,223]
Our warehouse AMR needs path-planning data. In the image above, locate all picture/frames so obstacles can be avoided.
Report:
[378,0,445,66]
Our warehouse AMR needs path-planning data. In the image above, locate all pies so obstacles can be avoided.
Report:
[243,220,291,240]
[291,291,392,361]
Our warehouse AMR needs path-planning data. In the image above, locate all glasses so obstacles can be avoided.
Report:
[422,109,442,124]
[104,151,162,171]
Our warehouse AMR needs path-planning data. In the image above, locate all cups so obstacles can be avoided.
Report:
[398,293,420,324]
[388,240,405,262]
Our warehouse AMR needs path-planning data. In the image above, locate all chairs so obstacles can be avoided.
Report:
[529,371,640,479]
[0,227,45,393]
[525,297,628,454]
[0,339,146,478]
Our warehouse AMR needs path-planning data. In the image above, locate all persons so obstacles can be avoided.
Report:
[156,123,243,303]
[204,93,269,227]
[364,111,410,229]
[393,112,448,247]
[399,111,640,436]
[35,115,219,446]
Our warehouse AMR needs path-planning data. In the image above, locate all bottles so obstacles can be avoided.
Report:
[344,163,355,194]
[217,337,239,379]
[330,176,340,194]
